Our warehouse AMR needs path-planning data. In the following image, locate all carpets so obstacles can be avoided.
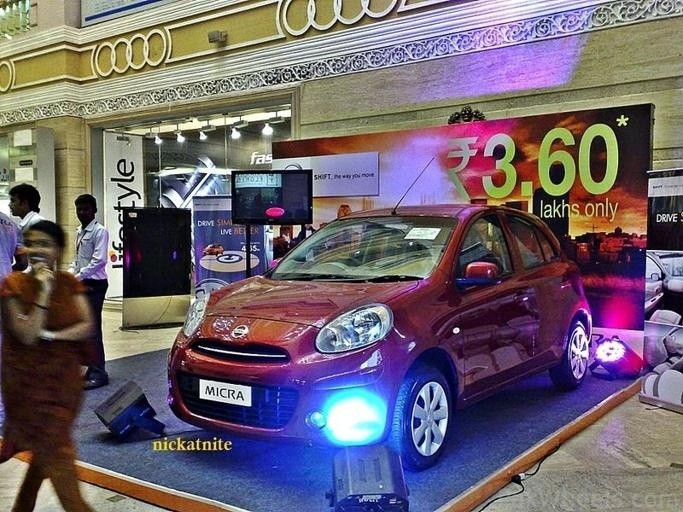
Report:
[0,346,648,511]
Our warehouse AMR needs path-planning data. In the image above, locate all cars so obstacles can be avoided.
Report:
[165,159,593,473]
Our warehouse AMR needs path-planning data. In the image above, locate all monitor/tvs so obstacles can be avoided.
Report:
[123,208,193,298]
[231,169,313,225]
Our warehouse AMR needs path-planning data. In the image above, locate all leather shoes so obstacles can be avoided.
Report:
[82,373,110,389]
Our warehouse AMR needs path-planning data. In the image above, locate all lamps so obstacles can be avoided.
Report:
[115,105,292,146]
[589,338,641,378]
[92,380,165,439]
[208,30,227,43]
[332,446,409,512]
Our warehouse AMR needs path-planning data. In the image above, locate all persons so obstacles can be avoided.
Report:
[0,221,97,512]
[0,212,29,445]
[9,184,56,275]
[233,188,355,263]
[65,192,110,389]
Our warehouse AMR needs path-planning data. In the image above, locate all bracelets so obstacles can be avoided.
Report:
[32,301,47,310]
[39,329,56,341]
